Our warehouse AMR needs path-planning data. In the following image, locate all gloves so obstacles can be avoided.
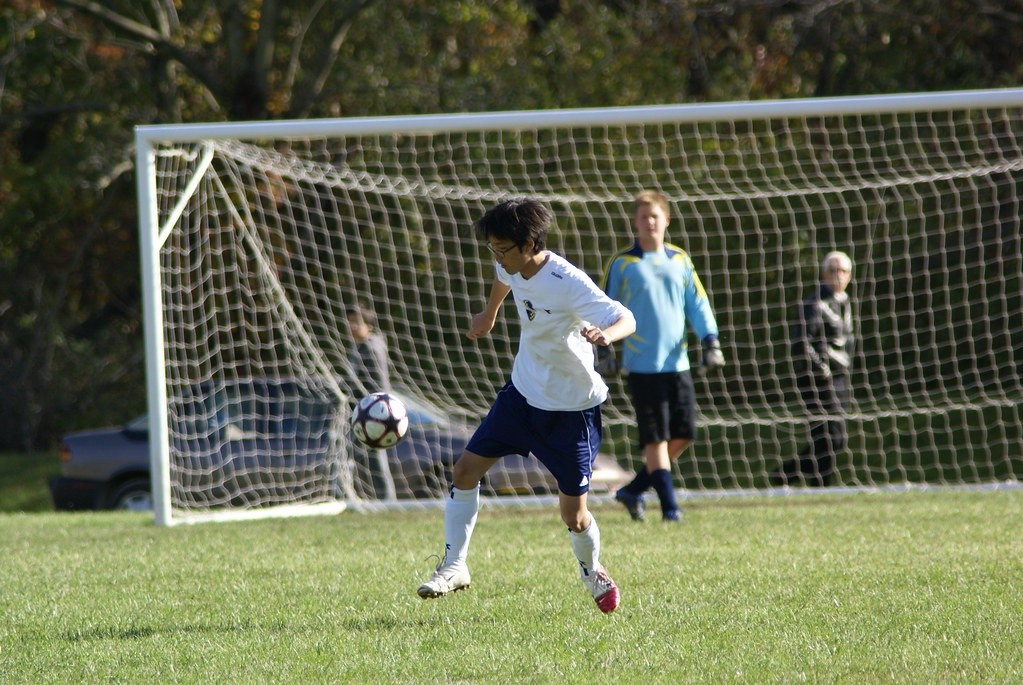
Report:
[706,338,726,371]
[594,343,618,379]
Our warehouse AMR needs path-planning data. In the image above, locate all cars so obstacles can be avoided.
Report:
[46,374,559,512]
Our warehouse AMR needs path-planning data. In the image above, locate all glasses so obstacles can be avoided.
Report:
[486,242,519,259]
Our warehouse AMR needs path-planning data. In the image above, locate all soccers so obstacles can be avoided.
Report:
[350,391,410,451]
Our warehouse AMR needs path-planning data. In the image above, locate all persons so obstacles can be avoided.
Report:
[326,310,399,499]
[597,187,729,526]
[417,196,637,615]
[770,248,856,488]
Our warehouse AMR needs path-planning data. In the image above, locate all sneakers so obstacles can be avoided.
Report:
[664,508,682,521]
[616,487,648,524]
[417,556,472,599]
[579,564,620,614]
